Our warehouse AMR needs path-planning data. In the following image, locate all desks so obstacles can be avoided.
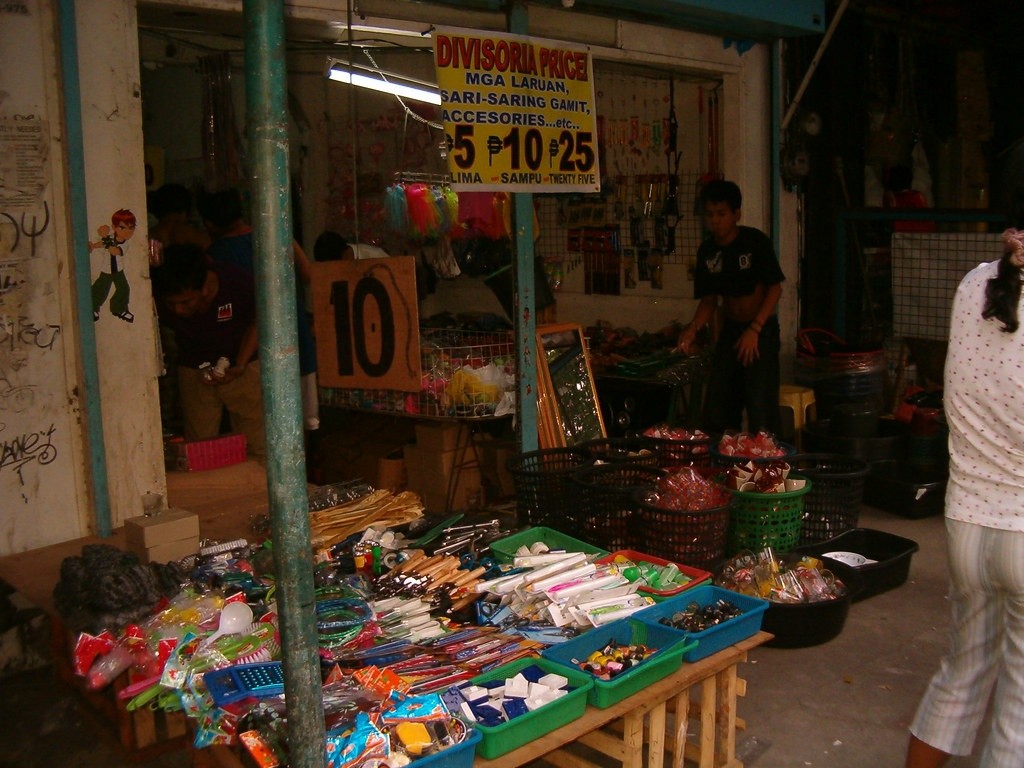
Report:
[0,481,320,739]
[241,628,775,768]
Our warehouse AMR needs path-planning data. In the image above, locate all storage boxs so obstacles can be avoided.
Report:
[122,421,773,768]
[794,521,923,607]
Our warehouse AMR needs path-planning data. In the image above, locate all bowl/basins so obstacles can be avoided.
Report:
[758,587,850,648]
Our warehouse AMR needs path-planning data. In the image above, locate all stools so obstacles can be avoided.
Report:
[739,384,818,468]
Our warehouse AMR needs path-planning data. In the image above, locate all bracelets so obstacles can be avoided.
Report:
[754,320,763,327]
[749,326,760,335]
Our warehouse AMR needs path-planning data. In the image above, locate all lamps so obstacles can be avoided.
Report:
[322,57,441,106]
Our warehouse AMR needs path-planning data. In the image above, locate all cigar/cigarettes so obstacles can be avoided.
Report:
[671,342,684,353]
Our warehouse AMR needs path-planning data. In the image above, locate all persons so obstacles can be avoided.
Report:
[677,180,786,437]
[314,231,391,261]
[147,183,328,456]
[907,186,1024,768]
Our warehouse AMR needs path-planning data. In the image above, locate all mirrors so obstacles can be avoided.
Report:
[508,322,610,469]
